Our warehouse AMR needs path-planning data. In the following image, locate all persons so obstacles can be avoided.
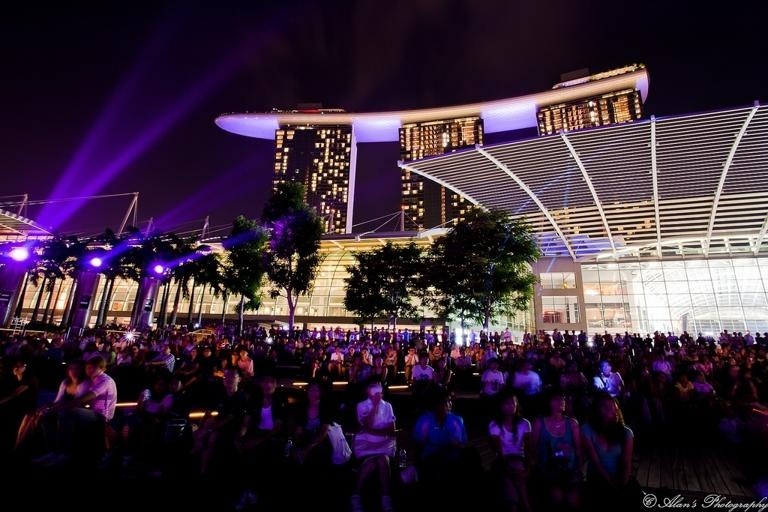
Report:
[1,325,768,512]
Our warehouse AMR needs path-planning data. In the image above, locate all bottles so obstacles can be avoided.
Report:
[398,447,408,469]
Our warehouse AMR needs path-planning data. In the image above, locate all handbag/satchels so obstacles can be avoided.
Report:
[327,421,351,465]
